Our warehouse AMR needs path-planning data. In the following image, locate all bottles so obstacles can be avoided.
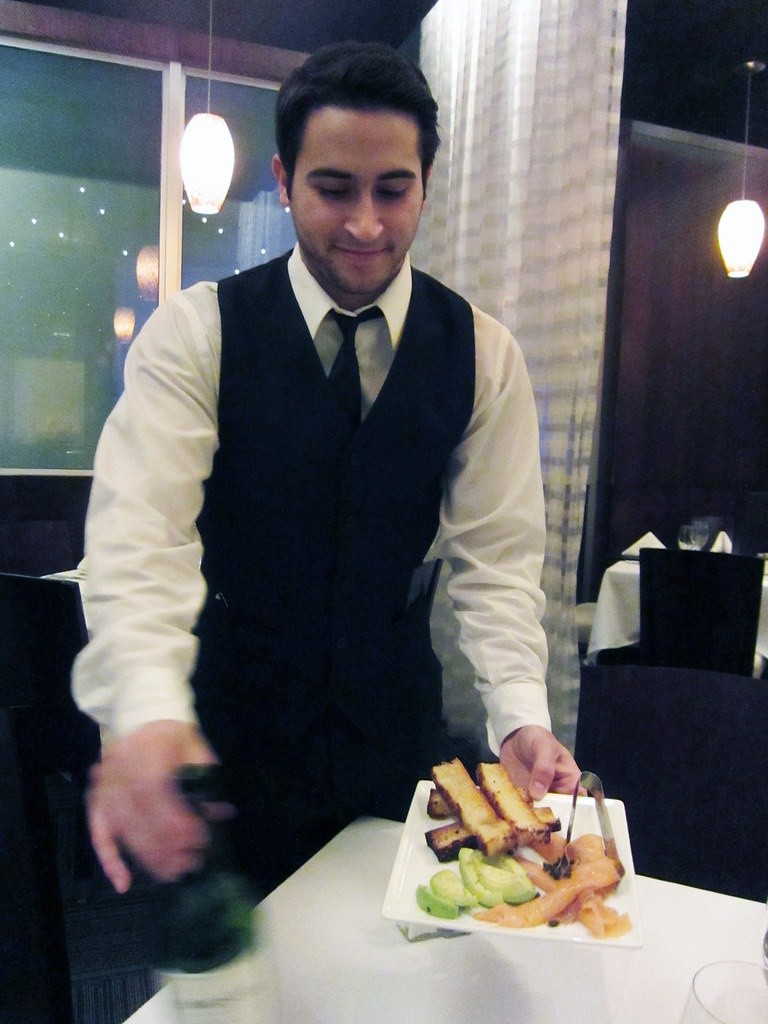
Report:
[146,764,262,1024]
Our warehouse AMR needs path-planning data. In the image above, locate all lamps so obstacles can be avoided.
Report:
[136,239,161,301]
[716,56,766,283]
[176,0,236,218]
[113,300,135,346]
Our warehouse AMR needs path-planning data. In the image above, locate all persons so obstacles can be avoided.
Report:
[72,47,590,896]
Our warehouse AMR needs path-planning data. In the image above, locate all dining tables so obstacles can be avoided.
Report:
[121,816,768,1024]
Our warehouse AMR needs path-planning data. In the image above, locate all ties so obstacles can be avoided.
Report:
[329,306,385,427]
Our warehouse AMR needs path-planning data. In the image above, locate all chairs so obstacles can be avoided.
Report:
[1,570,110,834]
[570,662,767,902]
[637,546,766,672]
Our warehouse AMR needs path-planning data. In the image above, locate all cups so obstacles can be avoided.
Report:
[678,960,768,1023]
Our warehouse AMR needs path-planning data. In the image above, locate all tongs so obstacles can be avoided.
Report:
[555,771,626,892]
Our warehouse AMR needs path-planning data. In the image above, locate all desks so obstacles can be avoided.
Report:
[585,534,767,664]
[39,559,92,639]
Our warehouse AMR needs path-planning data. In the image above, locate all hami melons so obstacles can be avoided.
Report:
[417,848,539,919]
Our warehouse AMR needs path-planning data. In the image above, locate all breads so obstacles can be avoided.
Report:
[424,757,562,863]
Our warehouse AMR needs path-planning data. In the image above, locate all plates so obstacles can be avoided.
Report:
[380,780,645,950]
